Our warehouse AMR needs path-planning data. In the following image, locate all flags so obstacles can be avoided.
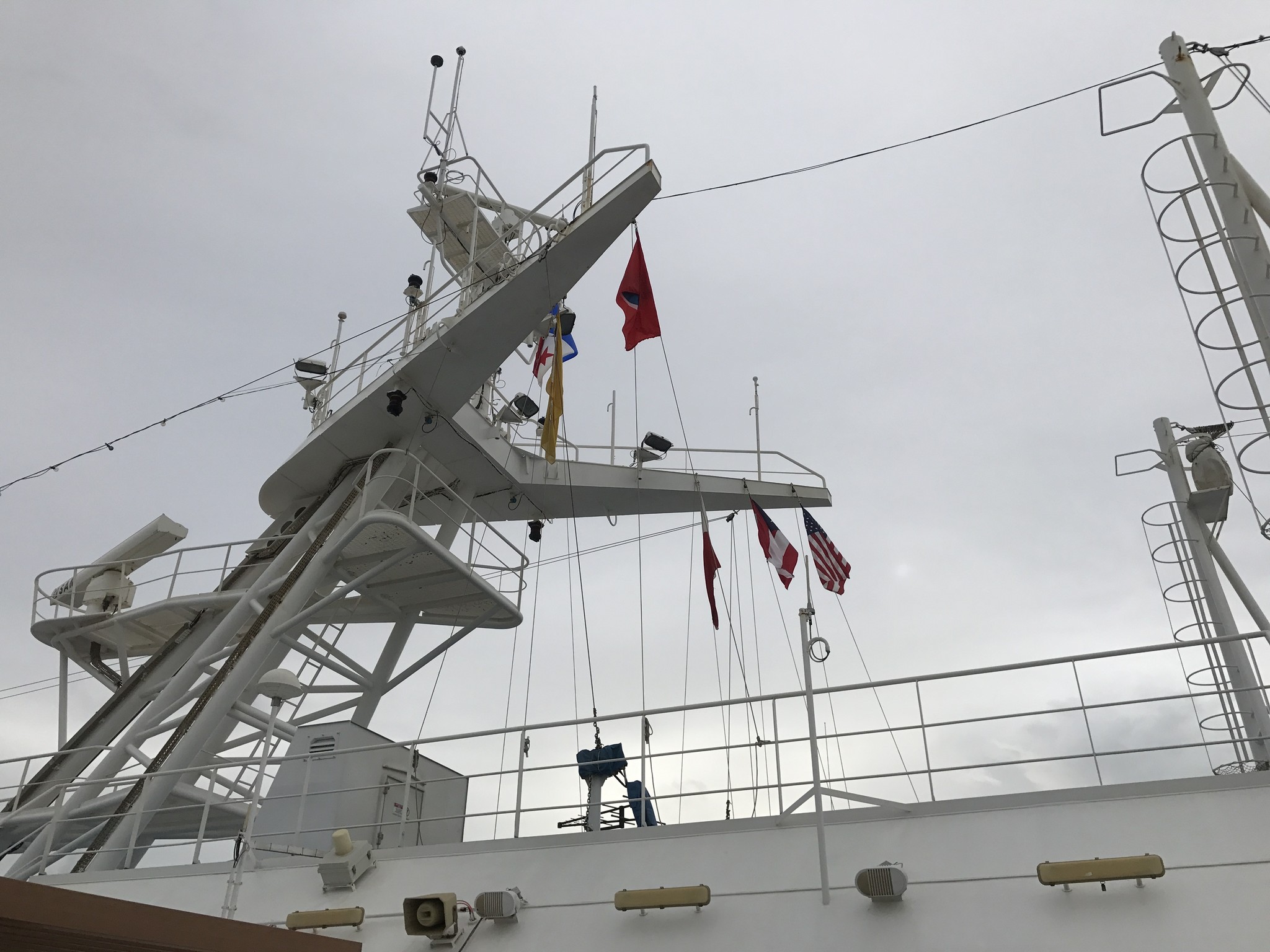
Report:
[746,495,797,590]
[531,302,579,389]
[615,226,661,352]
[799,504,851,596]
[699,491,723,633]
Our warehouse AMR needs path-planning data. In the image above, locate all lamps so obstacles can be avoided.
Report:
[386,385,407,417]
[528,520,544,543]
[640,431,674,457]
[295,357,328,381]
[509,392,539,420]
